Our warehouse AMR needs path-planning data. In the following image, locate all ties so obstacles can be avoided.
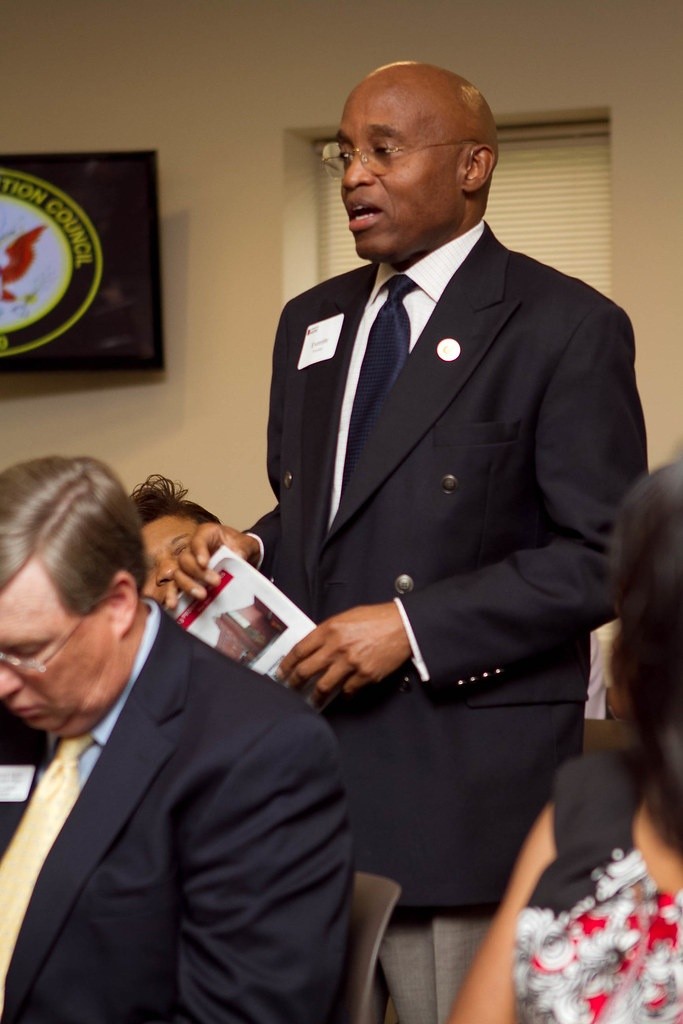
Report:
[0,733,94,1022]
[339,274,422,513]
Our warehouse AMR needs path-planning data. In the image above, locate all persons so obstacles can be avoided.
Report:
[163,58,651,1024]
[445,457,683,1024]
[0,456,357,1024]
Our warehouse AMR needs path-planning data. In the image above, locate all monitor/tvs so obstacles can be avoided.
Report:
[0,147,166,374]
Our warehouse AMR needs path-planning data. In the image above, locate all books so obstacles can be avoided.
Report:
[164,545,355,716]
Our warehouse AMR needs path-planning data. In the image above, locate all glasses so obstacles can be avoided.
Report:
[322,139,479,181]
[0,592,117,679]
